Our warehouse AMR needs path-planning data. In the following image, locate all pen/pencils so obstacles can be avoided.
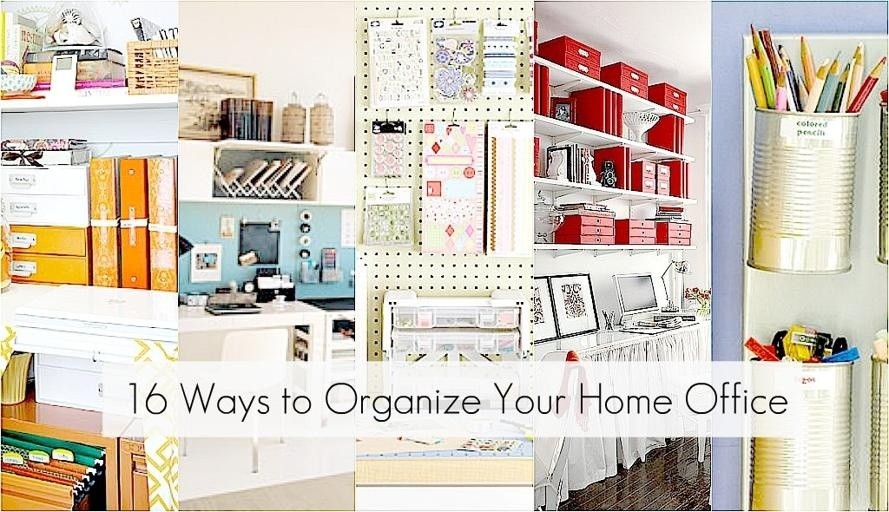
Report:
[746,24,887,113]
[601,310,615,331]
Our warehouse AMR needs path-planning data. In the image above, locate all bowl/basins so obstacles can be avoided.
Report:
[0,74,39,97]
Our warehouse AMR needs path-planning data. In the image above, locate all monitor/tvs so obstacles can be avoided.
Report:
[238,222,281,265]
[613,273,658,316]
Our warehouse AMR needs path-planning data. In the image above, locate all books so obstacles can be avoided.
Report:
[220,97,272,140]
[1,138,91,166]
[1,10,128,90]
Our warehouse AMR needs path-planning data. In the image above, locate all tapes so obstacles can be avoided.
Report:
[299,210,312,258]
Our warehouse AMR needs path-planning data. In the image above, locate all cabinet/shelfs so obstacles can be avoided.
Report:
[0,1,713,512]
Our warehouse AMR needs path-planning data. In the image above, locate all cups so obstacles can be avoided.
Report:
[2,351,31,406]
[275,294,286,306]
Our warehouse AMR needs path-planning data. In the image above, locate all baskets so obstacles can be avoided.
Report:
[127,39,179,95]
